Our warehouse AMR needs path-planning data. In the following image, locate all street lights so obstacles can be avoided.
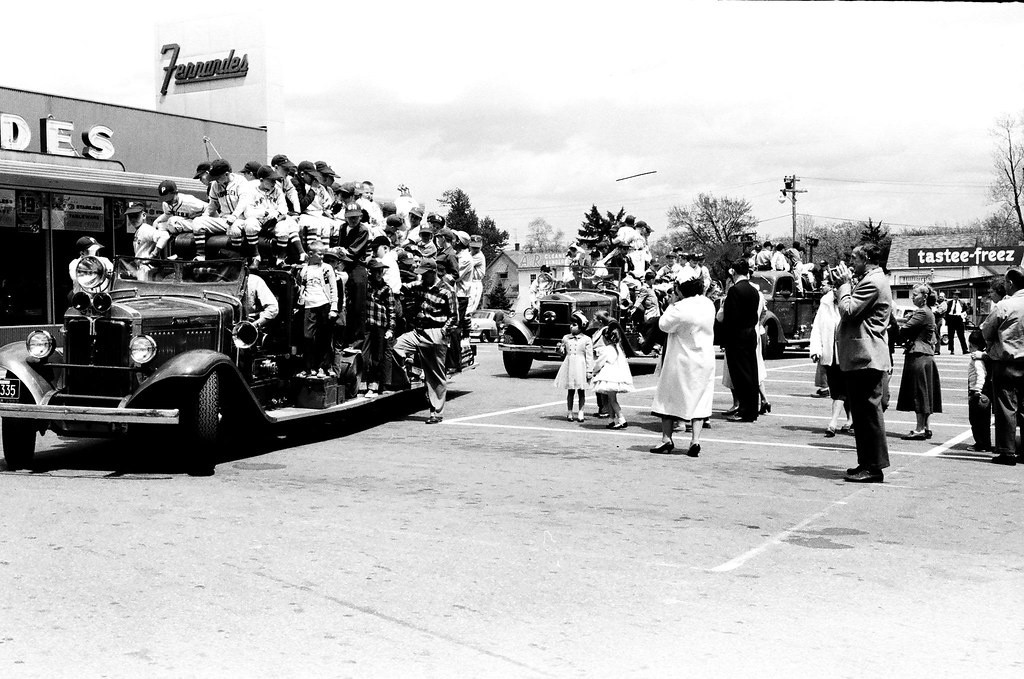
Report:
[779,195,797,245]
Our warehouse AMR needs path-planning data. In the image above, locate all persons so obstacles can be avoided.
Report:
[590,329,635,430]
[528,214,834,359]
[552,311,594,423]
[898,283,943,441]
[809,243,899,482]
[650,267,715,457]
[68,154,487,424]
[590,311,617,419]
[926,287,973,355]
[716,257,774,423]
[493,306,505,342]
[967,267,1024,466]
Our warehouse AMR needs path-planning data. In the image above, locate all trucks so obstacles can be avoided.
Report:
[497,266,725,379]
[750,270,825,359]
[0,243,476,466]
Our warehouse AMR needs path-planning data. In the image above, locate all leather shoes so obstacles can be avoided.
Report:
[426,417,443,424]
[844,466,883,483]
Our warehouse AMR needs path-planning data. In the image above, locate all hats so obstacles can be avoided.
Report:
[566,215,654,258]
[540,265,583,273]
[603,274,615,285]
[77,236,105,251]
[952,289,961,293]
[158,180,177,202]
[193,154,483,274]
[650,242,827,274]
[124,200,144,214]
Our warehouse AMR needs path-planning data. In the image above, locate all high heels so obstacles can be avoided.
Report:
[650,441,700,457]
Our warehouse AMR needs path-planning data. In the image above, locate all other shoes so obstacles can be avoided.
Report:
[567,413,712,433]
[168,254,310,271]
[722,402,771,422]
[357,375,424,398]
[344,347,362,354]
[446,369,462,374]
[825,424,932,441]
[296,368,336,378]
[934,351,971,355]
[967,444,1024,466]
[811,390,829,398]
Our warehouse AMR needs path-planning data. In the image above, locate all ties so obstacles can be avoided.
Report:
[953,301,957,314]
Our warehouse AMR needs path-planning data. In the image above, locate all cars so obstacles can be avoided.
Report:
[892,306,958,346]
[468,308,507,343]
